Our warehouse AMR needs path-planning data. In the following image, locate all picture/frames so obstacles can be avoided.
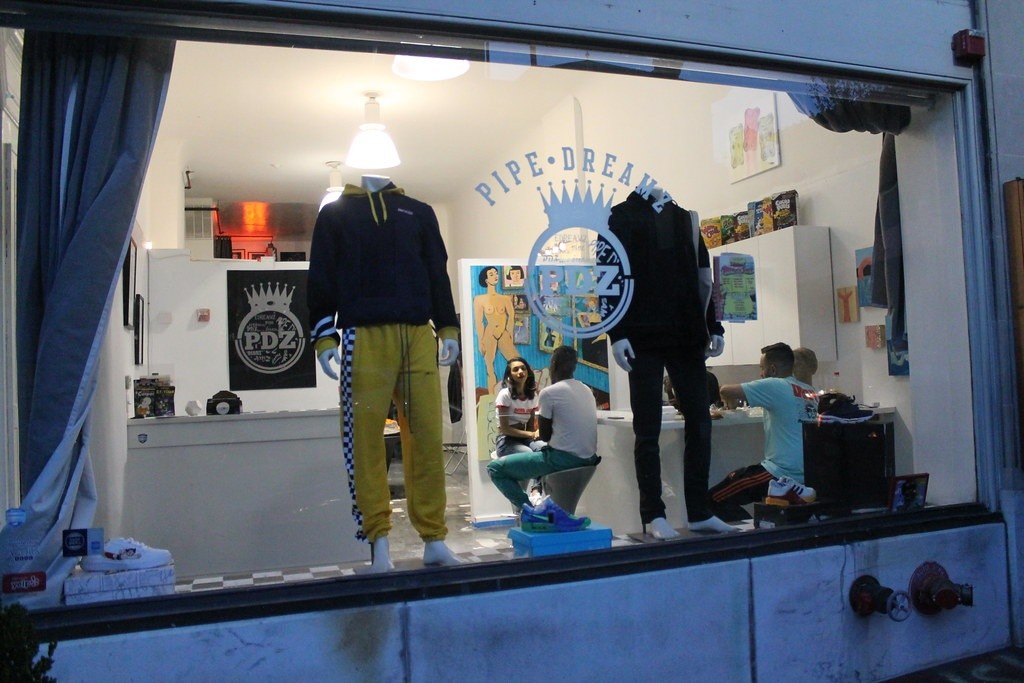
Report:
[134,293,144,364]
[122,235,138,327]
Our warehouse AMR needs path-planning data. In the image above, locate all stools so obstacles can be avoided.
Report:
[542,465,598,514]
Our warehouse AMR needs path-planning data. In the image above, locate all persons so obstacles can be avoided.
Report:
[707,342,820,522]
[793,347,817,399]
[596,186,739,539]
[494,357,547,507]
[487,345,597,517]
[306,174,460,575]
[663,371,721,407]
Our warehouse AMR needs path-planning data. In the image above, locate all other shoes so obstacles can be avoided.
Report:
[528,492,540,507]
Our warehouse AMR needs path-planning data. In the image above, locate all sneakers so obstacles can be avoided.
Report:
[520,494,592,533]
[766,475,816,506]
[80,537,171,571]
[817,393,875,424]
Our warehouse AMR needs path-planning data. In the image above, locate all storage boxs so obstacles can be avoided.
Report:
[754,498,848,527]
[508,521,612,558]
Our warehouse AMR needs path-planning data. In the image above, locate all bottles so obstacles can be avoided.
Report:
[1,508,40,578]
